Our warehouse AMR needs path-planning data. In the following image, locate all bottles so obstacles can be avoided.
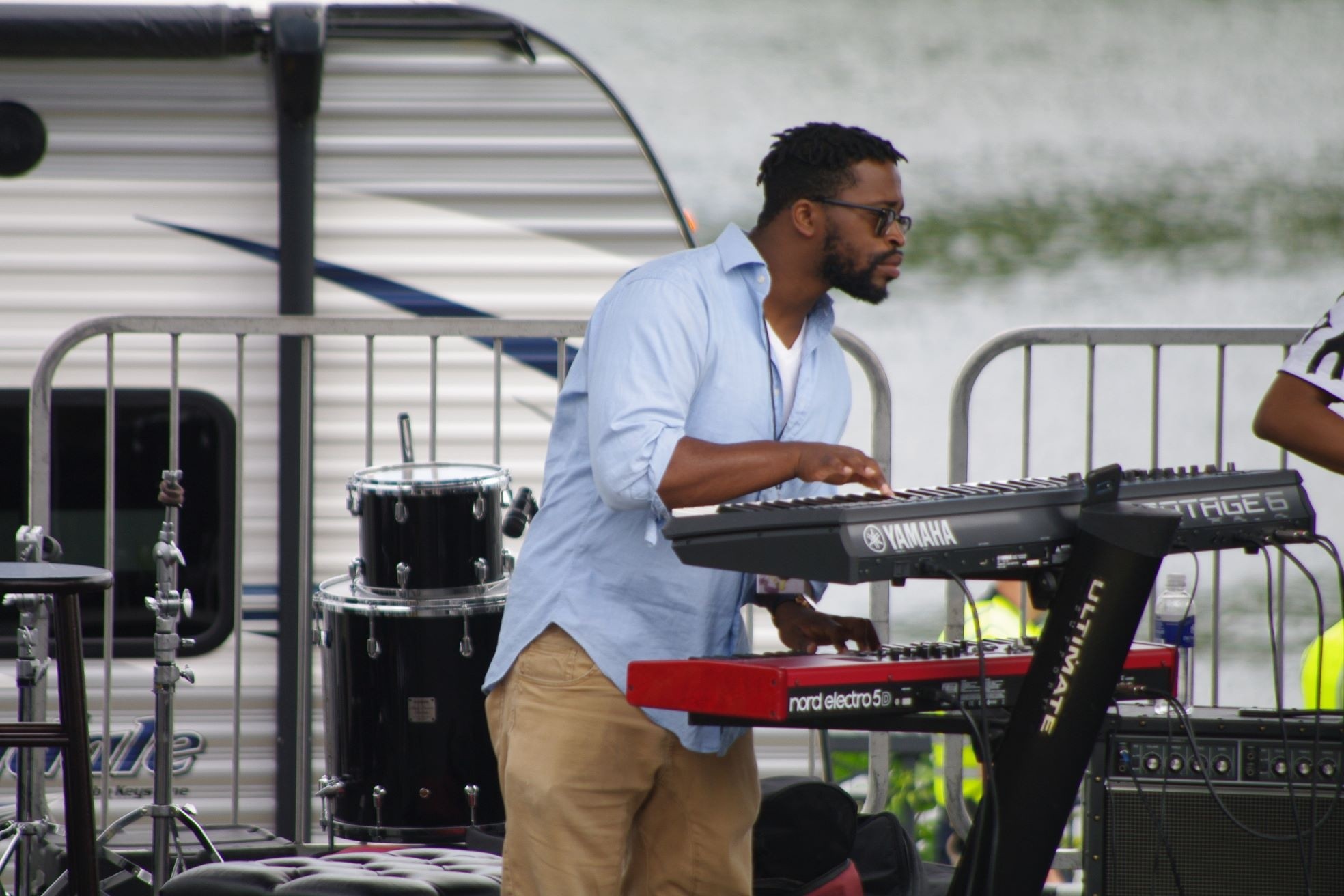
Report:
[1150,573,1196,715]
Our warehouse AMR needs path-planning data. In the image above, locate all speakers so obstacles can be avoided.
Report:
[1082,704,1343,896]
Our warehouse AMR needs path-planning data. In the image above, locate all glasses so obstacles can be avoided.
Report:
[816,200,911,237]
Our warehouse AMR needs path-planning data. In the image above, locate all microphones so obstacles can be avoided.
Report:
[1,101,46,176]
[501,486,532,539]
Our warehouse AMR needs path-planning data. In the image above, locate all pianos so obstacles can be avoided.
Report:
[624,461,1319,896]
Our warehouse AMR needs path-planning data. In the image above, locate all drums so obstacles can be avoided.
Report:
[310,460,517,842]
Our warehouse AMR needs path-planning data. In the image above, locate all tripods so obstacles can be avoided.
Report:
[0,472,225,896]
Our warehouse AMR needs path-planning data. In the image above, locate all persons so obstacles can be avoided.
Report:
[1252,291,1344,475]
[479,122,911,896]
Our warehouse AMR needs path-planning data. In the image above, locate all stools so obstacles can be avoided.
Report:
[0,561,118,896]
[159,846,505,896]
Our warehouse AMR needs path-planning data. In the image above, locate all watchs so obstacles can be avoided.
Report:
[771,593,811,613]
